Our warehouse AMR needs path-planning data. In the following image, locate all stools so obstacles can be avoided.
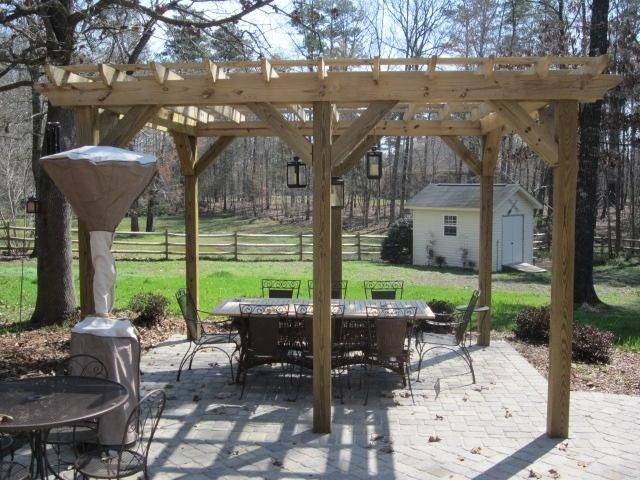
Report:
[455,304,489,348]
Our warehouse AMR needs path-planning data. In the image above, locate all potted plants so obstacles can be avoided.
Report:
[418,298,451,334]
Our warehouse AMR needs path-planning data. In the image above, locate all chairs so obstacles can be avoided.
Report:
[261,279,301,298]
[308,279,348,300]
[364,306,418,406]
[0,353,166,480]
[415,289,480,383]
[177,287,241,381]
[238,302,295,397]
[363,280,404,299]
[293,304,347,405]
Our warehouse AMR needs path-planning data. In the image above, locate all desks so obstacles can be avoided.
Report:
[209,296,436,376]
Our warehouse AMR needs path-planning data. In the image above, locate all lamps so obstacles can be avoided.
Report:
[367,126,382,178]
[27,197,39,213]
[287,156,307,187]
[330,176,344,209]
